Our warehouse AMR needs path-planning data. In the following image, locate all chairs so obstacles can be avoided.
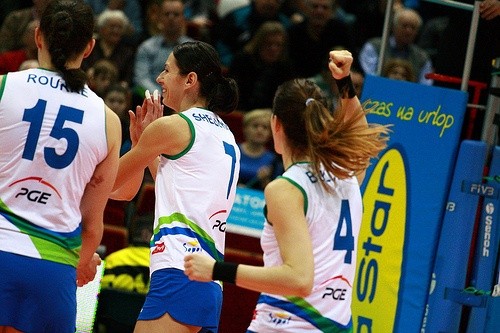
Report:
[100,182,264,333]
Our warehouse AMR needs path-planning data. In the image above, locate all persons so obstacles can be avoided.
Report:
[0,0,434,194]
[92,207,154,333]
[0,0,158,333]
[184,50,394,333]
[108,41,241,333]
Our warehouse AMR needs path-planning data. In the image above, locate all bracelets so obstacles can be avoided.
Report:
[336,74,356,101]
[211,259,241,284]
